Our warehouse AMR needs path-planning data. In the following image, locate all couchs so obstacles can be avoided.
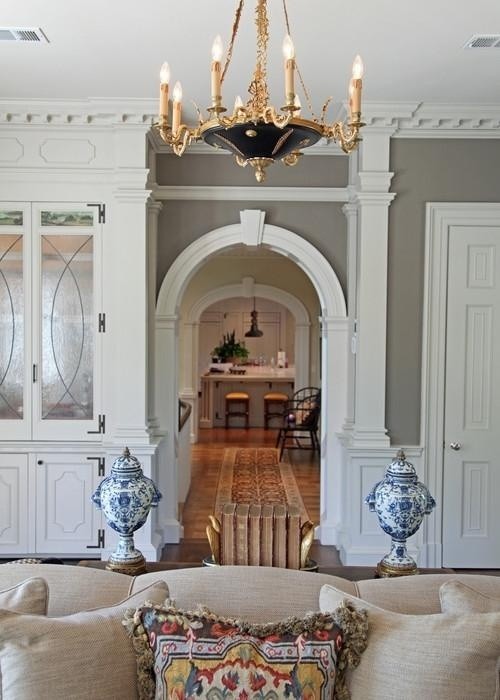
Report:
[0,563,500,698]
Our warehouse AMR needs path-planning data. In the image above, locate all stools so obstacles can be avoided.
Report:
[264,392,289,431]
[224,391,249,430]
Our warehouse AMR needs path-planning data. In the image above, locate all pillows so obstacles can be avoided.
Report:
[124,599,368,699]
[2,576,48,615]
[2,579,169,699]
[438,578,499,613]
[318,583,500,699]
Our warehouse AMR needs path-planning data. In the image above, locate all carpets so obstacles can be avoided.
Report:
[215,447,311,522]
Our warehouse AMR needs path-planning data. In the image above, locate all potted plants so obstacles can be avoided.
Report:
[210,328,250,366]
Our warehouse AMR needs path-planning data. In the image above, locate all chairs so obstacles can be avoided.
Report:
[276,387,320,463]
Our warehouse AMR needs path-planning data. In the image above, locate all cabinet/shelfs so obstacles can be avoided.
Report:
[1,199,107,559]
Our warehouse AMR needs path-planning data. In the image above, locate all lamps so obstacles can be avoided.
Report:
[244,297,264,337]
[152,0,365,184]
